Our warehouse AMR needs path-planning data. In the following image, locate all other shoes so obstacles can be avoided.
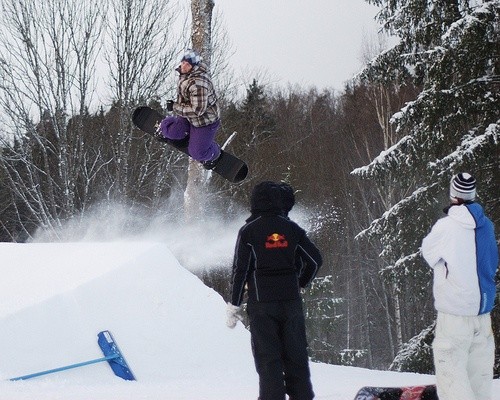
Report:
[203,149,222,169]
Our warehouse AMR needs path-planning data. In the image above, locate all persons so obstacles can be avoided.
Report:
[160,49,223,170]
[227,181,323,400]
[421,173,500,400]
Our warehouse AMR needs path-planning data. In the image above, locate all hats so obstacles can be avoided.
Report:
[181,48,201,66]
[449,172,477,200]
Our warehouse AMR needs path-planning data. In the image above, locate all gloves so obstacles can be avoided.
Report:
[225,302,241,328]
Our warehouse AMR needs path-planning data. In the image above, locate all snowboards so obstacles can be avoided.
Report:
[354,384,439,400]
[131,106,248,183]
